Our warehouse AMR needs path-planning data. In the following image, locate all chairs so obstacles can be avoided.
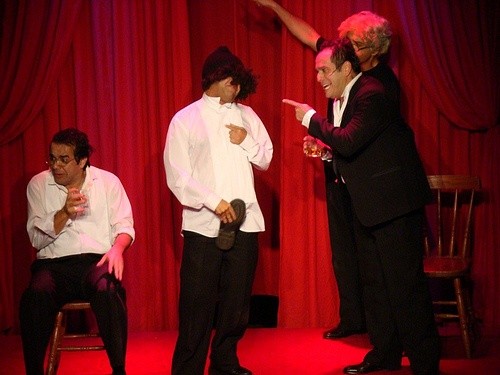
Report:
[407,173,484,360]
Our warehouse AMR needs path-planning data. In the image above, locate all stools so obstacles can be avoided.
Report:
[45,302,107,375]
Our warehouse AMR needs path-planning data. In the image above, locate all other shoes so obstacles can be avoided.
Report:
[216,198,247,251]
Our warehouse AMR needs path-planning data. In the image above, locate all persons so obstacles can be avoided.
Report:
[257,0,401,338]
[18,126,134,375]
[163,47,273,375]
[282,43,441,375]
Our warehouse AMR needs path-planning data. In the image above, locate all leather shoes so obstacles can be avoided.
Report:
[208,363,253,375]
[344,357,387,374]
[324,320,368,338]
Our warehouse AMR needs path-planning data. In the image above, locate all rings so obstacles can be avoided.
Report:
[74,207,76,211]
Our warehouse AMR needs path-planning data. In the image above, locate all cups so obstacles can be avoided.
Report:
[306,133,322,156]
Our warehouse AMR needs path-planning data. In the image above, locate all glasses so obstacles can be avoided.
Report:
[312,66,342,79]
[349,38,369,51]
[43,152,75,170]
